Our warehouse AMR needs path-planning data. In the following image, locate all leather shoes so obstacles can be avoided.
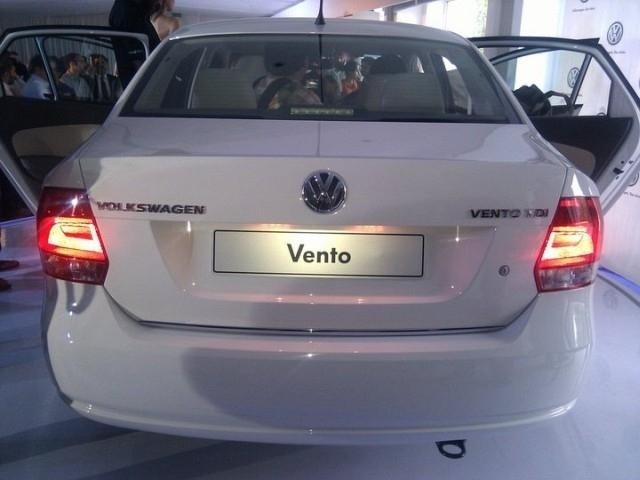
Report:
[0,278,12,292]
[0,260,19,271]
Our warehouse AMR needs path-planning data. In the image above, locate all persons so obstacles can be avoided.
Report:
[253,50,405,110]
[109,0,182,114]
[0,51,124,289]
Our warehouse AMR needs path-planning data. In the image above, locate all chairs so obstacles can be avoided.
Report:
[191,67,257,110]
[354,70,444,112]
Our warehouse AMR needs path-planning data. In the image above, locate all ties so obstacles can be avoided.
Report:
[100,76,109,99]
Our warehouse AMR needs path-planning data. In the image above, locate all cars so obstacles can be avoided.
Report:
[3,0,640,457]
